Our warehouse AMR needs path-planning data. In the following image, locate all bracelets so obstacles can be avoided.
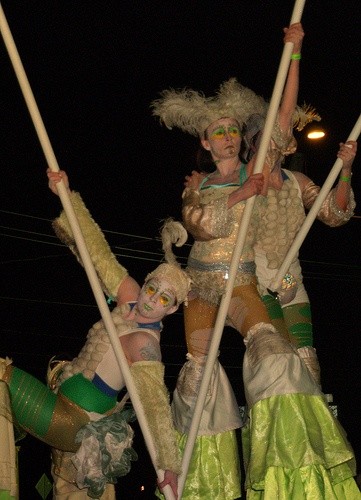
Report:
[340,172,353,182]
[293,46,301,51]
[290,54,301,60]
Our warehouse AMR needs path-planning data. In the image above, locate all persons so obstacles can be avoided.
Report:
[0,166,191,500]
[169,23,361,500]
[184,129,358,390]
